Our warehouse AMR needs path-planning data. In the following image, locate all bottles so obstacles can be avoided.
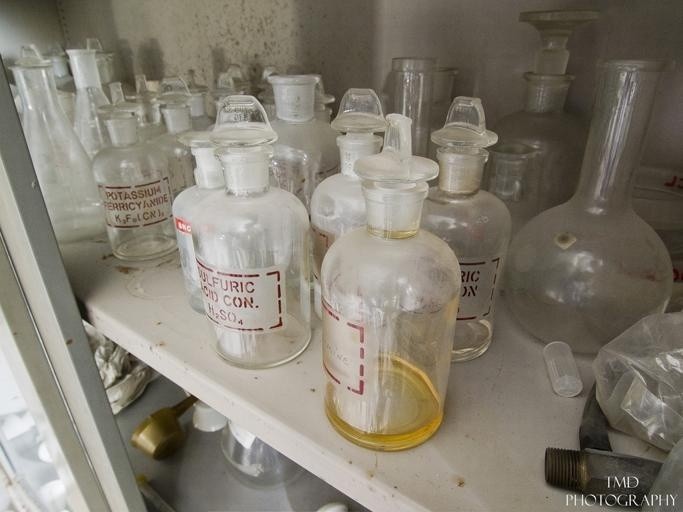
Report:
[508,54,680,358]
[9,39,580,361]
[324,182,464,453]
[197,148,315,369]
[219,418,306,489]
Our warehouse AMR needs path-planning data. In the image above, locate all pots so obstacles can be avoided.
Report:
[129,393,198,460]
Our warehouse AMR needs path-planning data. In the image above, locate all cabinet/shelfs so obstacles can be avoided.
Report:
[0,0,683,512]
